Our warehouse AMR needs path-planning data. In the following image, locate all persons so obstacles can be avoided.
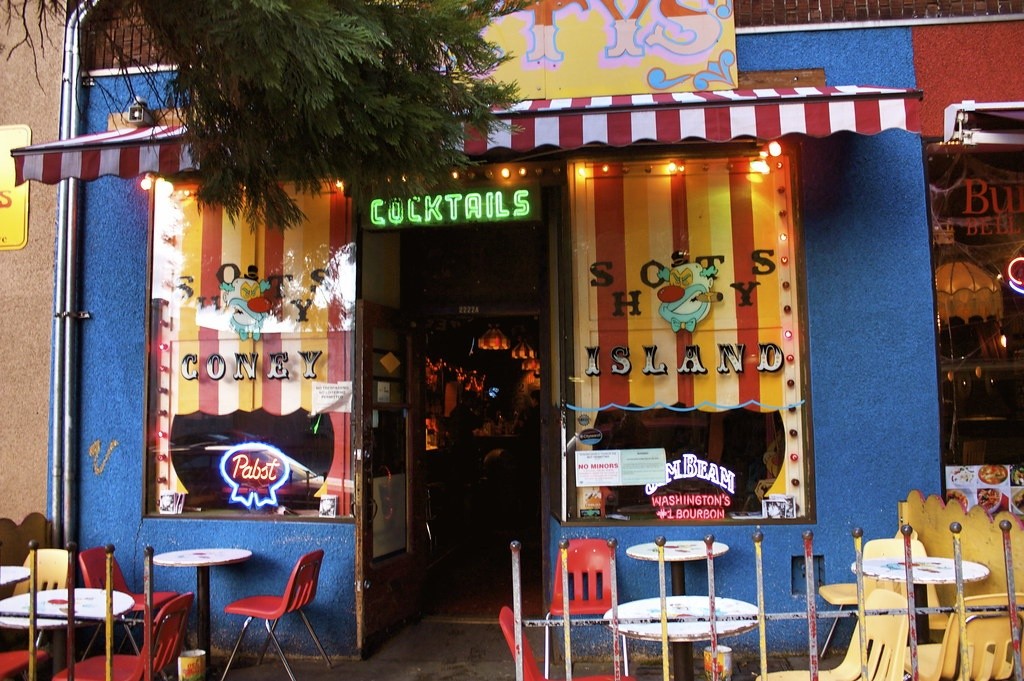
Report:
[445,390,488,459]
[526,390,540,422]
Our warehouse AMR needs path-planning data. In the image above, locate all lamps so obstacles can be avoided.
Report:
[934,246,1003,328]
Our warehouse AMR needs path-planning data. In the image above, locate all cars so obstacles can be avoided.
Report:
[595,419,709,458]
[169,443,355,515]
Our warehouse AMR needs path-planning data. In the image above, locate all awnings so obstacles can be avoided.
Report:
[9,82,922,190]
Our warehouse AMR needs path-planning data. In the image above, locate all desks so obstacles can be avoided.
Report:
[850,556,991,646]
[0,587,136,679]
[151,548,254,677]
[603,595,761,681]
[626,540,730,597]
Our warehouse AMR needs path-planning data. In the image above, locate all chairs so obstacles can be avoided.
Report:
[498,606,639,681]
[753,527,1024,681]
[545,539,629,678]
[0,547,68,681]
[79,546,180,659]
[220,549,332,681]
[51,591,194,681]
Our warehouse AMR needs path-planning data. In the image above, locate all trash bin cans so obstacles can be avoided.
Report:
[703,644,733,681]
[176,648,207,681]
